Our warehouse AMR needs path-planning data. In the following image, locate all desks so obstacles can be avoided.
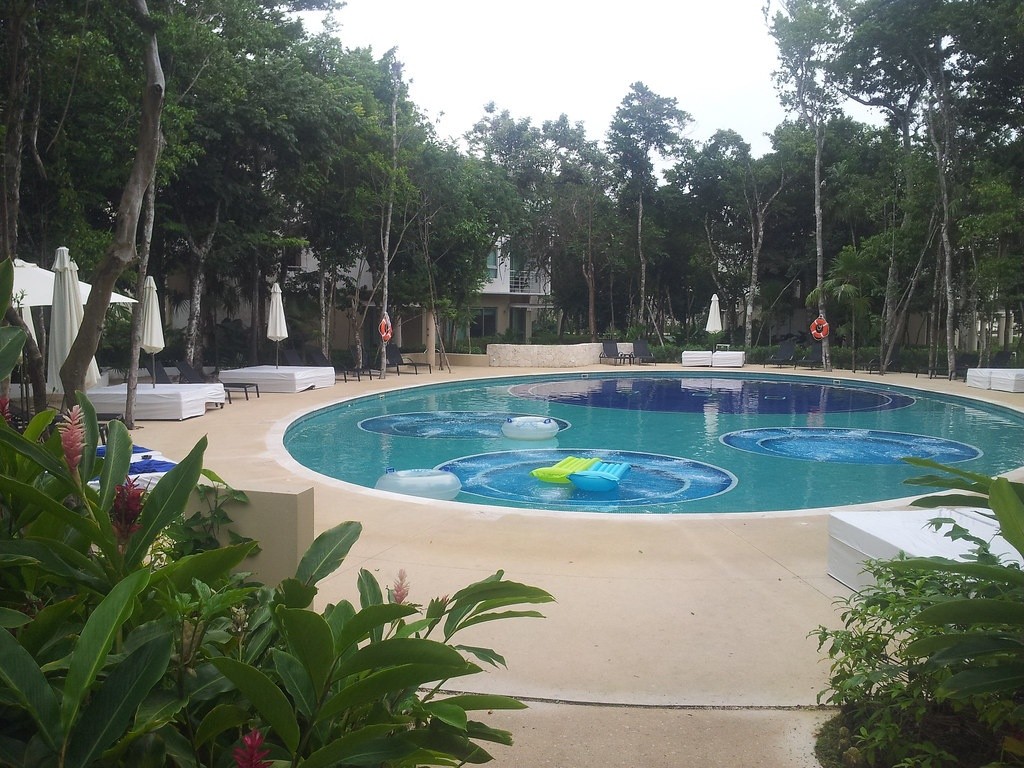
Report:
[712,379,743,389]
[620,353,634,365]
[682,378,712,390]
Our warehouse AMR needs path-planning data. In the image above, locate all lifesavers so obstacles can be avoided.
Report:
[379,319,393,342]
[373,465,461,501]
[810,318,829,340]
[500,415,559,442]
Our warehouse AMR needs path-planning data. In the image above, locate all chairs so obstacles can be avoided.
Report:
[794,342,822,371]
[283,349,347,384]
[600,380,617,396]
[349,345,381,378]
[949,351,1013,383]
[869,343,902,375]
[306,346,372,382]
[164,348,260,401]
[599,339,631,366]
[762,342,796,370]
[386,344,431,376]
[140,351,231,408]
[915,352,979,379]
[630,340,656,367]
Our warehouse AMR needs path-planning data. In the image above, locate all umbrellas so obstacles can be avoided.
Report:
[704,293,722,354]
[268,282,289,368]
[9,305,40,425]
[139,275,167,389]
[6,259,141,426]
[45,245,102,394]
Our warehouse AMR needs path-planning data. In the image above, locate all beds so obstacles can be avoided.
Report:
[826,507,1024,664]
[88,383,226,421]
[681,350,712,367]
[712,350,745,368]
[82,443,180,493]
[967,368,991,390]
[220,365,336,392]
[991,368,1024,393]
